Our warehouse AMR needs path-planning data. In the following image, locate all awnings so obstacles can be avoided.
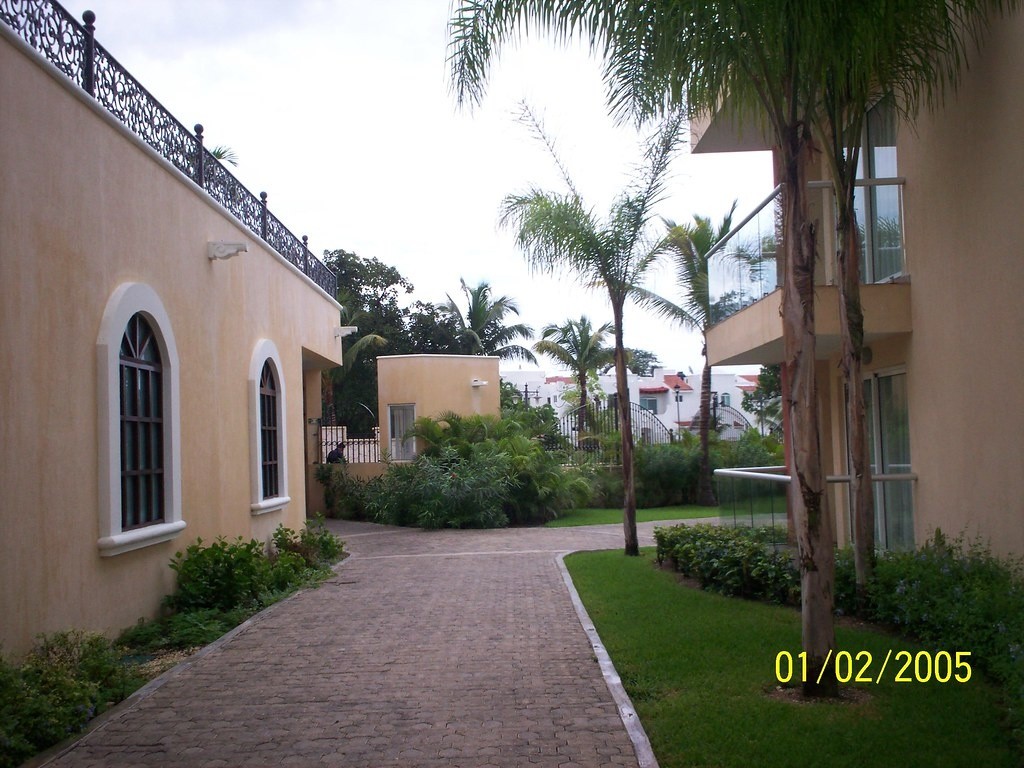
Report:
[639,388,669,393]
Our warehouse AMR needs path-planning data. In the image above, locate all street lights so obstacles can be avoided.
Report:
[674,383,681,440]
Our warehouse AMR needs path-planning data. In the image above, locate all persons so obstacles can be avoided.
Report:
[327,443,345,463]
